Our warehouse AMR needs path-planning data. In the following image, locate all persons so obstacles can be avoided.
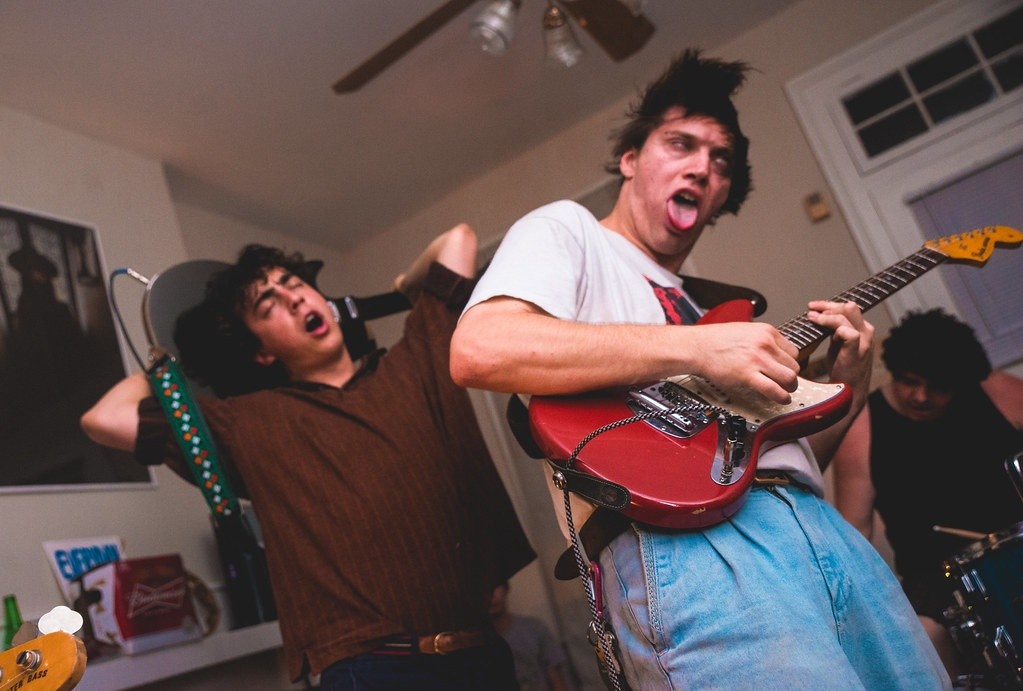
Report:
[834,307,1023,664]
[450,48,954,691]
[81,224,538,691]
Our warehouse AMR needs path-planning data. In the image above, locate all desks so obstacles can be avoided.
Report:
[69,618,309,691]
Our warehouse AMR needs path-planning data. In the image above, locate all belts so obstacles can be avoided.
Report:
[372,620,497,654]
[554,470,817,580]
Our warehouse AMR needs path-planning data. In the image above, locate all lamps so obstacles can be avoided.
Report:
[470,0,586,76]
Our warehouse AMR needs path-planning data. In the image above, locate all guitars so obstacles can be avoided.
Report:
[141,259,491,400]
[527,225,1023,529]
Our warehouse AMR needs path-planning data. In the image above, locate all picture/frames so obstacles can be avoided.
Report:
[0,202,160,495]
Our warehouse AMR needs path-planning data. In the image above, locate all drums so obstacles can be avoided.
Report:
[940,520,1023,691]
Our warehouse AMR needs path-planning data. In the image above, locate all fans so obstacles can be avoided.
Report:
[329,0,656,95]
